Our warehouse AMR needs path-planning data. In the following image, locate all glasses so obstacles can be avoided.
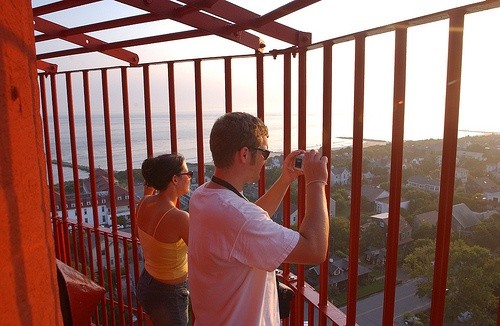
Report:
[178,170,194,178]
[247,147,270,160]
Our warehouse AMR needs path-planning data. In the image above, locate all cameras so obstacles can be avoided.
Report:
[293,154,306,171]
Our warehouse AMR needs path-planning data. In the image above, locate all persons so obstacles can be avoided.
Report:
[135,154,194,326]
[188,112,329,326]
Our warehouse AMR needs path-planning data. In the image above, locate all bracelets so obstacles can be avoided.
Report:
[306,179,327,186]
